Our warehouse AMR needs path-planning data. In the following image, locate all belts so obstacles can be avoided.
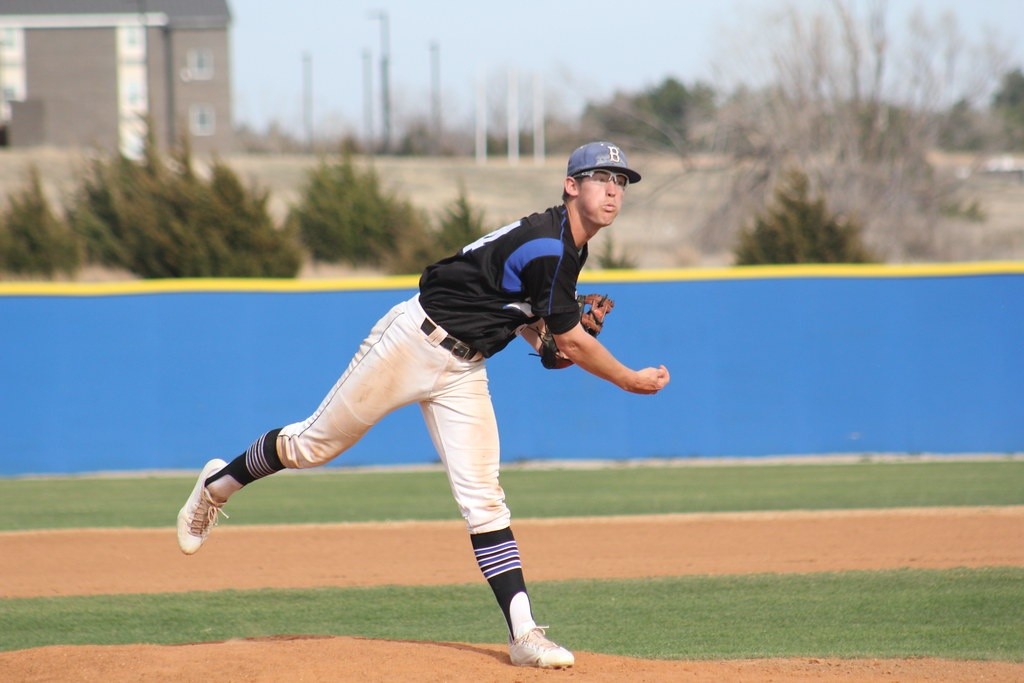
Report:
[420,318,483,361]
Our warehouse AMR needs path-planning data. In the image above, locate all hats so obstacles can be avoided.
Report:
[567,142,641,183]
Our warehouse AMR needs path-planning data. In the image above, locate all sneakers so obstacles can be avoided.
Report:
[177,458,229,555]
[508,626,575,667]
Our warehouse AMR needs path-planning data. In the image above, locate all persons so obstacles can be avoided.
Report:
[175,141,670,668]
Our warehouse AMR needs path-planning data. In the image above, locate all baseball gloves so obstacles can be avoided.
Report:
[526,292,615,370]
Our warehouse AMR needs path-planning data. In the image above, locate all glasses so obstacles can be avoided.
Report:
[572,168,629,188]
